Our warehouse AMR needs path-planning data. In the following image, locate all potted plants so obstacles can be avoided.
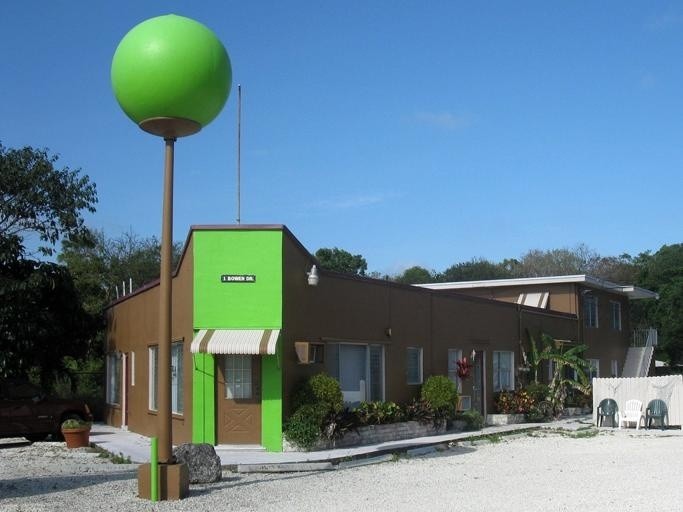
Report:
[61,417,93,448]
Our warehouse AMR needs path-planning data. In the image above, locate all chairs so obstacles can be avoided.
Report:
[644,398,669,430]
[618,400,644,429]
[596,398,618,428]
